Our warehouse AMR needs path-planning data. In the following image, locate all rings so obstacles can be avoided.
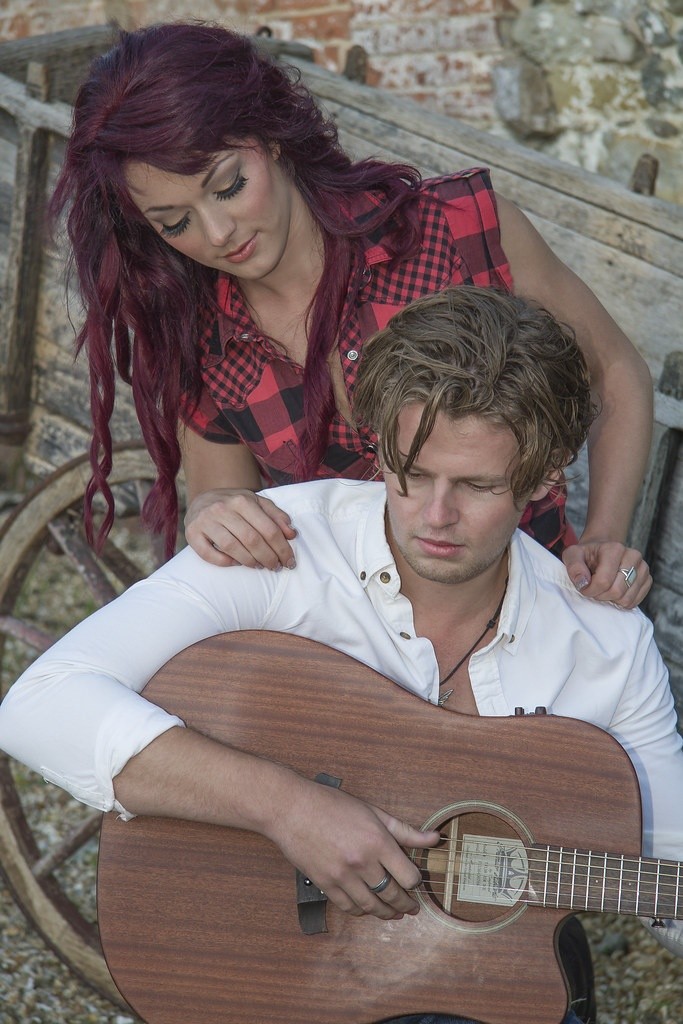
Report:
[370,870,391,893]
[617,566,638,588]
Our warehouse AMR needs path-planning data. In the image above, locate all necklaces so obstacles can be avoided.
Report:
[438,574,510,706]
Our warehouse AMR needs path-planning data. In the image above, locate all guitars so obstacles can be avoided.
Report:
[98,628,682,1024]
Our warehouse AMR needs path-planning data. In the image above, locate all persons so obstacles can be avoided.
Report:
[0,291,683,1024]
[43,25,654,610]
